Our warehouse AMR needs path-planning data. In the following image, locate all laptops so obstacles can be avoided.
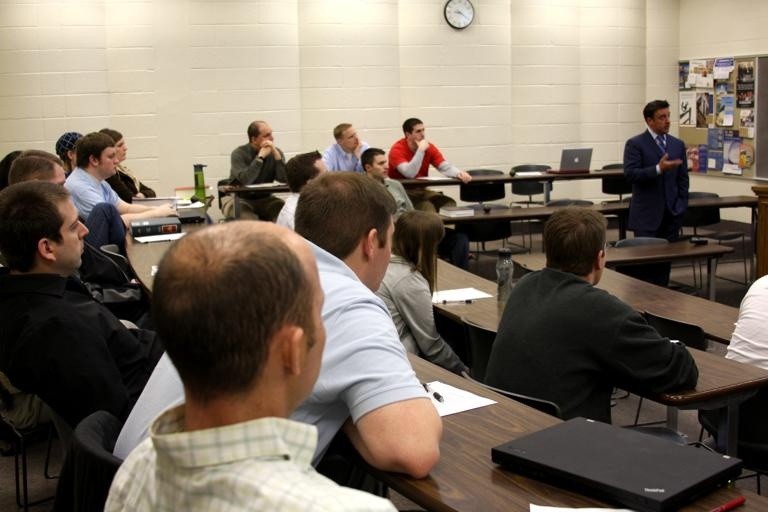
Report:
[546,148,593,173]
[491,415,743,512]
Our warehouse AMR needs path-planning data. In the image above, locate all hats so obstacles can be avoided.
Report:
[55,132,84,156]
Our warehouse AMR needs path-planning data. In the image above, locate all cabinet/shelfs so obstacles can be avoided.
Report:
[678,54,768,181]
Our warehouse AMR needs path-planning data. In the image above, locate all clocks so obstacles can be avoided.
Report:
[444,0,475,30]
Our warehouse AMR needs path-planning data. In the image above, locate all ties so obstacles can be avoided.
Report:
[656,135,667,154]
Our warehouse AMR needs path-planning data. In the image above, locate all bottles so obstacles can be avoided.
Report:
[193,162,206,201]
[494,248,515,303]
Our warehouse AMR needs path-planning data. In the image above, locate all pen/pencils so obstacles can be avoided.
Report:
[419,379,445,404]
[433,299,477,306]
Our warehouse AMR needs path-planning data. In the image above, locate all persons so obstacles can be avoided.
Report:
[724,273,768,444]
[484,203,717,452]
[104,222,399,512]
[623,100,689,287]
[223,120,468,374]
[113,172,442,477]
[0,128,164,424]
